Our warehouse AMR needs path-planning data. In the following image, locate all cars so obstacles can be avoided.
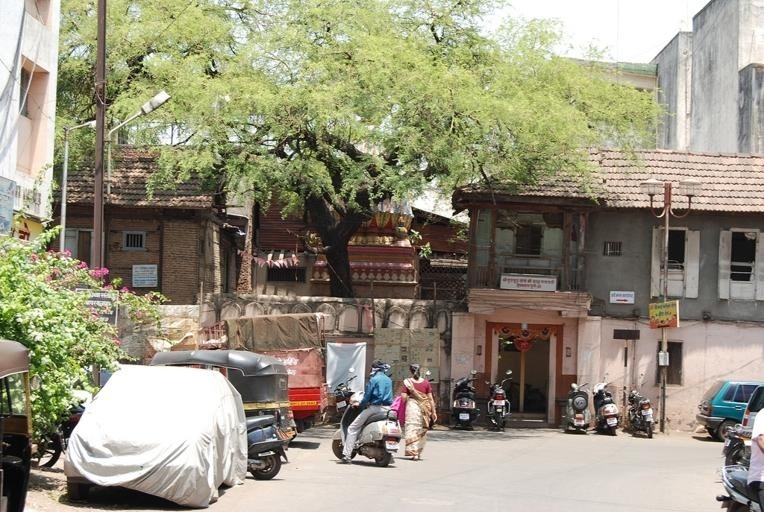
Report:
[696,378,764,441]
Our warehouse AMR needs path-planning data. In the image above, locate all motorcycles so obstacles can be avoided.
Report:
[247,414,288,479]
[720,424,753,471]
[713,464,764,512]
[447,368,655,439]
[332,375,401,469]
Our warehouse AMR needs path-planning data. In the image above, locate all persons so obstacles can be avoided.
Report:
[399,363,437,460]
[334,359,393,464]
[748,409,759,499]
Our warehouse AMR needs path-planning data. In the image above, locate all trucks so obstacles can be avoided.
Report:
[169,312,329,430]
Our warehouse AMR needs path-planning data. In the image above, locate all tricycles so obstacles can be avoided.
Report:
[148,350,295,453]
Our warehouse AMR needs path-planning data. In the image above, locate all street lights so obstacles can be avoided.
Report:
[58,89,172,255]
[639,178,700,434]
